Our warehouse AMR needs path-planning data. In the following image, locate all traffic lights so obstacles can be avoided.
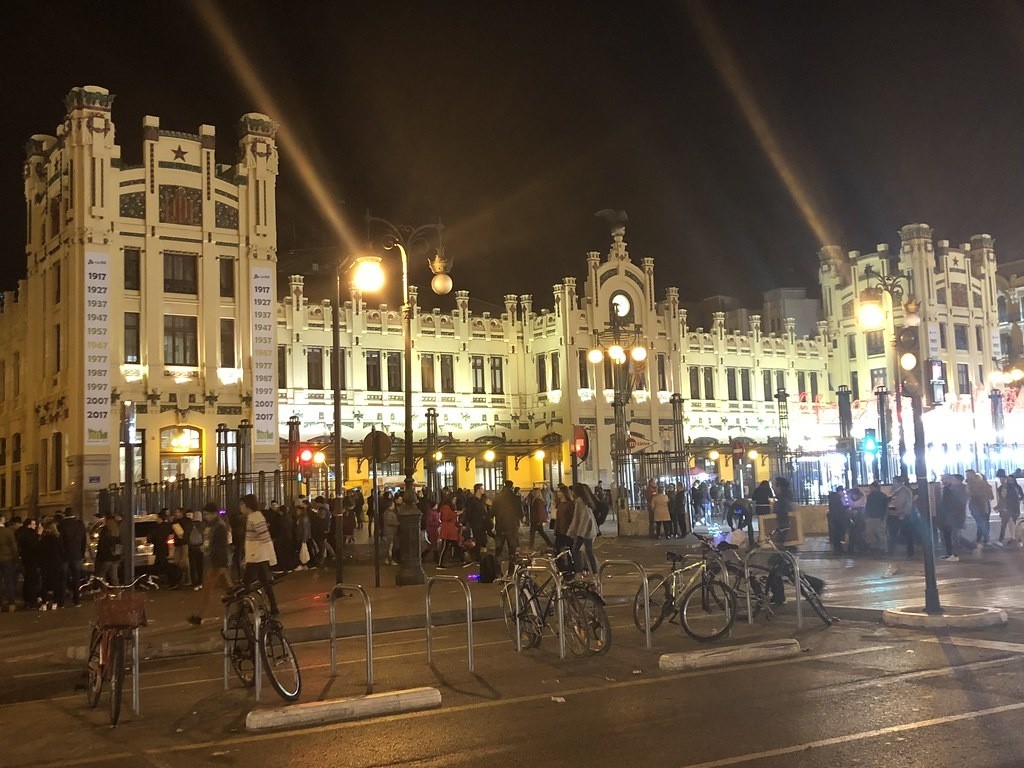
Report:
[300,445,313,468]
[899,325,925,400]
[865,427,877,455]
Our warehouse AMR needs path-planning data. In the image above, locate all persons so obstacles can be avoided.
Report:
[236,494,280,615]
[183,503,236,624]
[826,468,1024,561]
[0,475,794,613]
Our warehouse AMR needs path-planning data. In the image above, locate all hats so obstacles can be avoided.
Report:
[206,503,217,512]
[64,508,75,516]
[725,529,746,549]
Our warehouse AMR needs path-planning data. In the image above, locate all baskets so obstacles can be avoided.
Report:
[94,591,145,628]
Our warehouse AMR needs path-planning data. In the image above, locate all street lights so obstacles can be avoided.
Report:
[858,263,944,618]
[350,212,452,582]
[586,306,647,538]
[332,253,384,596]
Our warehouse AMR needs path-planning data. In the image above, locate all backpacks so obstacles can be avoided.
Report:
[189,527,202,545]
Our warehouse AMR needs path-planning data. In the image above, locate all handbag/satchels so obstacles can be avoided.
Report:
[566,523,575,538]
[479,554,501,582]
[300,544,311,565]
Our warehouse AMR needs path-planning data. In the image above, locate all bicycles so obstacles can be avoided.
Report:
[221,567,304,703]
[706,524,834,628]
[631,531,741,642]
[500,546,613,657]
[77,573,160,727]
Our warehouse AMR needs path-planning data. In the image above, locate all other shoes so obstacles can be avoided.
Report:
[945,555,958,562]
